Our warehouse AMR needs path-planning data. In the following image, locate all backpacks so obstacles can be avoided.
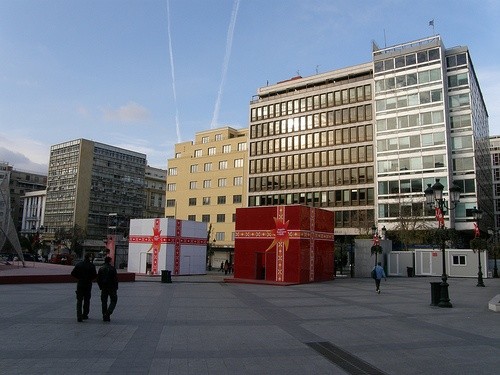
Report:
[371,266,377,279]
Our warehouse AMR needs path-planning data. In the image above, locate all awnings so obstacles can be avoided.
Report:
[77,240,106,246]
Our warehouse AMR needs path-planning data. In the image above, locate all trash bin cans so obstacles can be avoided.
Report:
[429,281,449,306]
[161,270,171,283]
[406,267,414,277]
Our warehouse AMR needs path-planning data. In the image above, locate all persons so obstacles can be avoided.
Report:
[372,262,387,294]
[218,260,232,274]
[71,253,97,322]
[98,257,118,321]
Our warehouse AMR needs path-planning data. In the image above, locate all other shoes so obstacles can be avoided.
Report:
[103,314,107,322]
[83,315,89,320]
[77,317,83,322]
[106,315,111,322]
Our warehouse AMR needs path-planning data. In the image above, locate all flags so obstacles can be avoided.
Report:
[429,20,434,25]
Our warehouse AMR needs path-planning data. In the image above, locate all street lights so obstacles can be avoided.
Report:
[423,179,461,308]
[371,224,386,266]
[471,206,485,287]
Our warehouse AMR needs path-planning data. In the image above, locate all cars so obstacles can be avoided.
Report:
[22,253,73,265]
[89,257,105,266]
